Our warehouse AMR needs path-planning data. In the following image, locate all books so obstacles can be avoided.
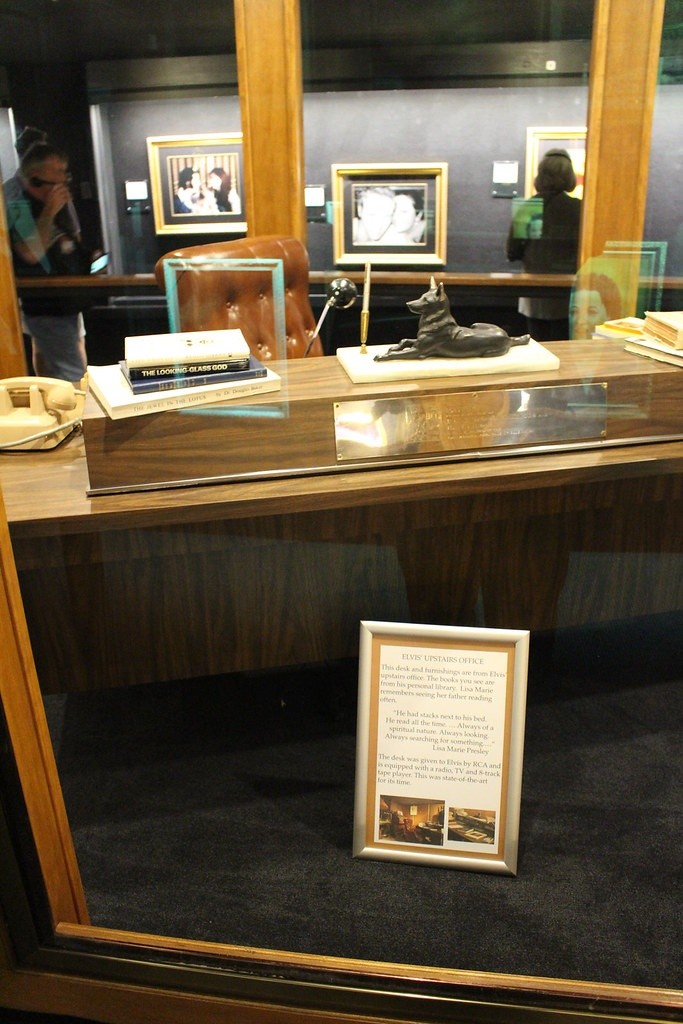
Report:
[592,306,683,365]
[83,330,280,422]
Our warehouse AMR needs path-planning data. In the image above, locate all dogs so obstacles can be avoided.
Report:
[373,275,530,362]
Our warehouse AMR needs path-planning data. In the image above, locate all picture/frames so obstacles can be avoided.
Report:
[526,126,590,202]
[560,240,670,423]
[147,133,248,235]
[163,255,289,421]
[330,164,448,269]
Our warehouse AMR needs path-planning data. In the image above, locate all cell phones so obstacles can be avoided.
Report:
[89,251,112,274]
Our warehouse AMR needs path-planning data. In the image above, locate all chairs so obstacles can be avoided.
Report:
[153,231,324,360]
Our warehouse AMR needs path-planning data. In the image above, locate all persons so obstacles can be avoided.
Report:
[571,271,623,339]
[176,162,243,215]
[354,185,428,248]
[11,146,90,378]
[507,148,581,341]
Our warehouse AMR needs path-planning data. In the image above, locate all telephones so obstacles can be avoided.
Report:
[0,375,86,450]
